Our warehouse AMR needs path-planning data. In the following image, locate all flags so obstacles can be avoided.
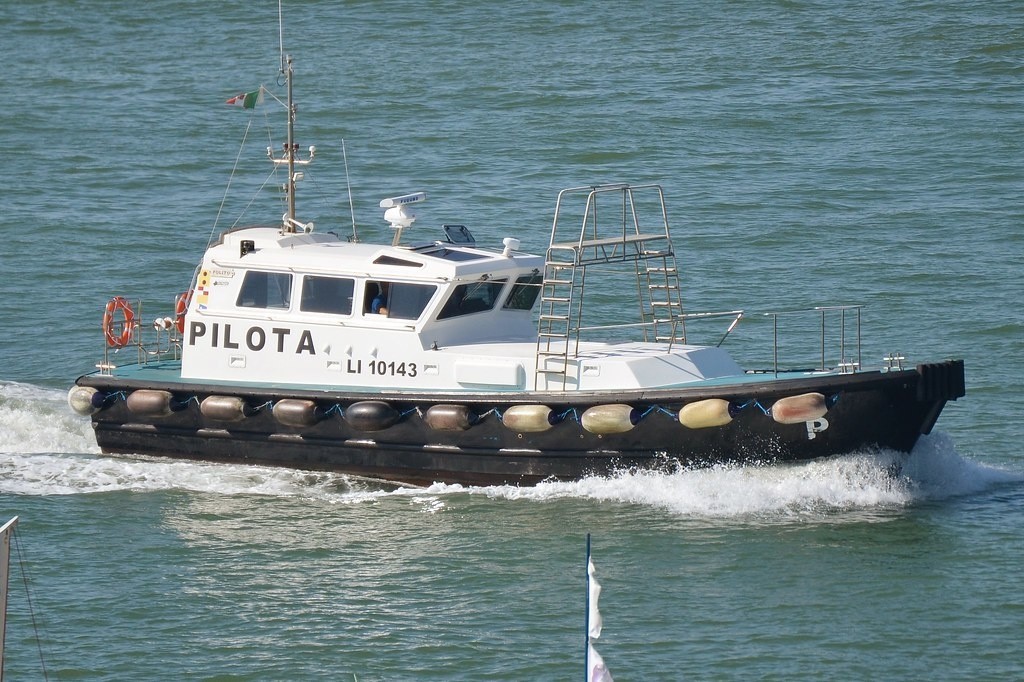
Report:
[588,558,602,638]
[587,644,614,682]
[226,87,263,109]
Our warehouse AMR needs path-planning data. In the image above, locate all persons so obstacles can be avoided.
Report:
[371,282,400,315]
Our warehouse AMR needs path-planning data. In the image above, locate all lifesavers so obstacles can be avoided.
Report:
[103,295,135,349]
[175,289,194,334]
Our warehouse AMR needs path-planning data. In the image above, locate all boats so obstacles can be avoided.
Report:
[66,1,967,505]
